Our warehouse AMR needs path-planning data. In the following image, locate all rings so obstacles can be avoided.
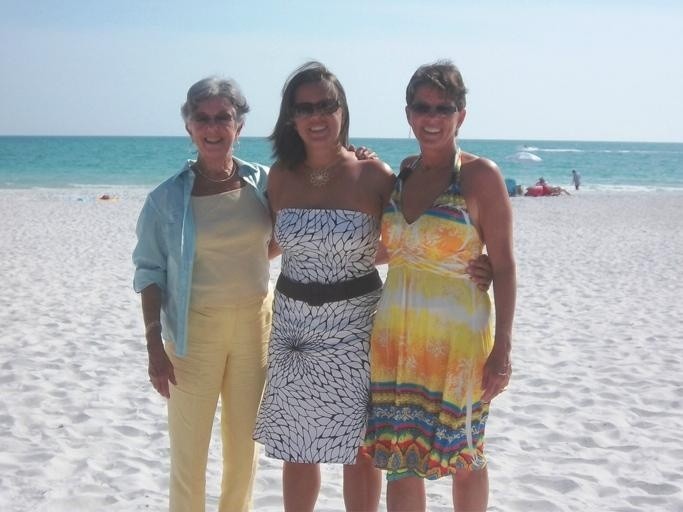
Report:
[500,388,507,392]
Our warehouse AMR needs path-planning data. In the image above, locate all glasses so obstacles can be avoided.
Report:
[187,110,235,124]
[409,101,458,116]
[288,98,342,117]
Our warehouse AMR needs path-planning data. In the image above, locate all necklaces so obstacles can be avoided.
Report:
[307,158,342,188]
[193,161,238,183]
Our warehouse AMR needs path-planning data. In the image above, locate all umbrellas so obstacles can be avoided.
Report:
[501,152,541,164]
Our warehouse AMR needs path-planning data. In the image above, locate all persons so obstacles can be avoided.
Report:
[263,58,493,510]
[134,72,378,511]
[359,61,516,511]
[537,176,573,196]
[570,168,582,191]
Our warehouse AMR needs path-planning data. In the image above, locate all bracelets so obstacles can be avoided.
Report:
[144,320,161,338]
